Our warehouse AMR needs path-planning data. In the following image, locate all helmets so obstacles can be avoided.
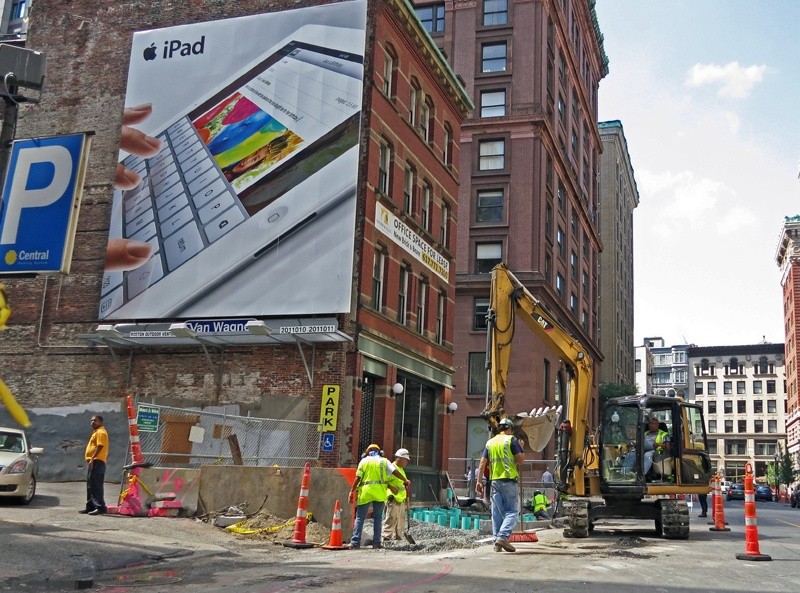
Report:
[534,490,540,492]
[497,418,514,429]
[394,448,410,460]
[365,443,380,455]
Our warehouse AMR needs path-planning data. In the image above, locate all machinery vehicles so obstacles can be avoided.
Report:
[478,261,712,540]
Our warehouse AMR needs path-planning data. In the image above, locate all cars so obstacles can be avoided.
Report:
[790,483,800,508]
[725,484,745,501]
[0,427,45,505]
[754,485,773,501]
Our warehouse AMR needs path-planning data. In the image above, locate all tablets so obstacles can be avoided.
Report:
[98,24,365,319]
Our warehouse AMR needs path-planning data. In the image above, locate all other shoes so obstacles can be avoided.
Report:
[373,545,383,548]
[495,538,516,552]
[698,514,707,517]
[343,543,356,548]
[494,546,502,552]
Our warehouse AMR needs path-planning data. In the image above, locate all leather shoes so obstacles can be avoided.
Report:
[89,509,107,515]
[78,509,89,514]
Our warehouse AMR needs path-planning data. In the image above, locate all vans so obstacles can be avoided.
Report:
[721,481,732,494]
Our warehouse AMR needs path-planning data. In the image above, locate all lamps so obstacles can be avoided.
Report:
[245,320,315,346]
[390,382,404,397]
[96,324,141,351]
[168,323,223,349]
[447,402,458,414]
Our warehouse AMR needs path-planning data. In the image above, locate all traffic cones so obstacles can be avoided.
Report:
[320,500,351,550]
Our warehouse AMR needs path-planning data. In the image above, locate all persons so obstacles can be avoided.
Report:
[698,494,708,517]
[103,103,161,272]
[541,467,553,488]
[221,134,289,183]
[475,418,524,552]
[342,444,410,549]
[464,466,478,497]
[617,419,671,481]
[531,490,550,520]
[78,415,108,515]
